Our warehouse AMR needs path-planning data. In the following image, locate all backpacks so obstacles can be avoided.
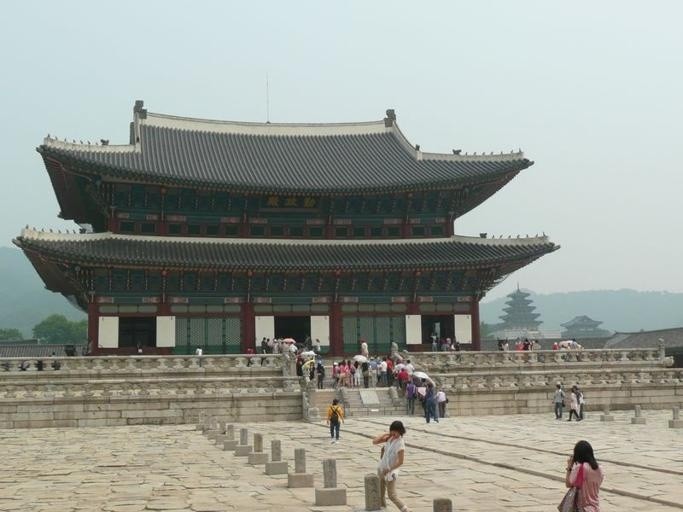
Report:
[330,406,339,425]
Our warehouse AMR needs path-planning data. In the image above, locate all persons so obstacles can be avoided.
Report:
[136,340,144,355]
[405,371,449,423]
[550,384,565,420]
[492,334,583,364]
[195,345,202,356]
[360,340,367,356]
[428,331,454,351]
[332,353,414,388]
[571,385,579,418]
[566,388,581,422]
[564,440,603,512]
[315,362,324,388]
[578,390,584,419]
[372,421,411,512]
[325,399,344,445]
[454,341,462,362]
[246,346,254,367]
[257,334,320,379]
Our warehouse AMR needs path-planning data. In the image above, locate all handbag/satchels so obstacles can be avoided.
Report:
[558,463,584,512]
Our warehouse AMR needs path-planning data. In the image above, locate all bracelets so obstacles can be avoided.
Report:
[565,468,571,471]
